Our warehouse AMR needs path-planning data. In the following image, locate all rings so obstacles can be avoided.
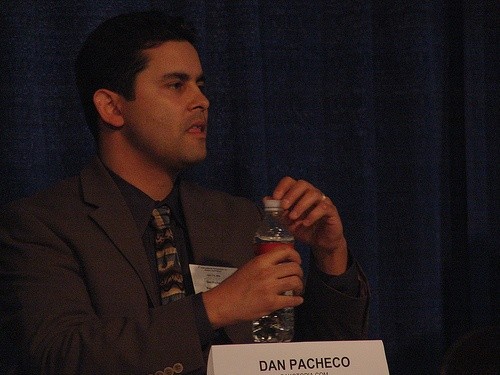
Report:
[322,193,326,202]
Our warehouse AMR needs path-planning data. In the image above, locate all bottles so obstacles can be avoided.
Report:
[251,198,296,345]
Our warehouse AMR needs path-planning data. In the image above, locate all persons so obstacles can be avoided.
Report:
[0,16,378,374]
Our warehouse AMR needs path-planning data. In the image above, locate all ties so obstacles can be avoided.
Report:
[150,203,187,308]
